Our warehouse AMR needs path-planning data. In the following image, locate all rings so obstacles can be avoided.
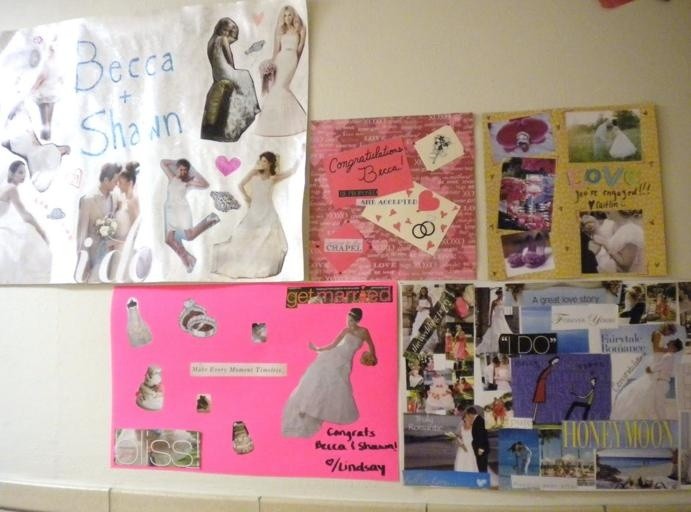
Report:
[179,298,208,329]
[187,315,220,340]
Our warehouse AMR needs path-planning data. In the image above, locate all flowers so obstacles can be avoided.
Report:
[360,351,377,366]
[92,215,119,240]
[443,431,470,452]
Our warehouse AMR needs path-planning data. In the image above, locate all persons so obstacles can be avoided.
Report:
[157,156,222,274]
[645,336,685,419]
[486,356,516,395]
[260,5,307,141]
[200,17,262,143]
[72,160,125,285]
[587,210,647,272]
[507,444,523,476]
[206,150,304,280]
[614,328,669,418]
[1,160,55,284]
[467,407,491,472]
[518,440,532,476]
[592,117,612,159]
[654,287,671,319]
[284,306,379,431]
[454,412,481,472]
[107,160,142,285]
[609,118,641,157]
[621,284,645,320]
[410,286,435,339]
[485,287,519,353]
